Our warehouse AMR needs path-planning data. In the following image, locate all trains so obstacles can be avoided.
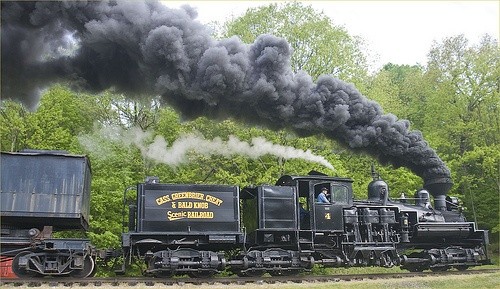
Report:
[0,147,494,280]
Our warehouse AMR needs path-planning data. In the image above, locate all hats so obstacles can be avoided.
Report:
[322,188,328,191]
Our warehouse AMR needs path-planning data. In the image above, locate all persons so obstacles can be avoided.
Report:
[317,188,329,203]
